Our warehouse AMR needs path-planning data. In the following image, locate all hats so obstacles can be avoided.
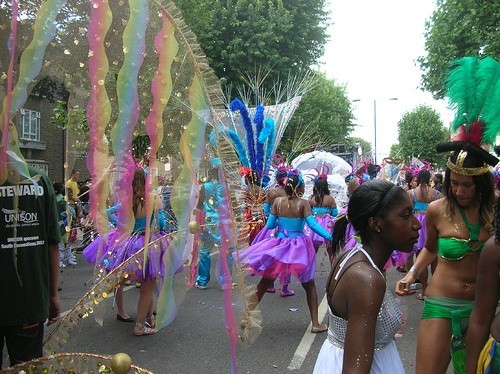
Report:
[435,56,500,177]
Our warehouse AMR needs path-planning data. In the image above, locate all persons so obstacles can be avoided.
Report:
[194,168,237,289]
[313,179,422,374]
[465,194,500,374]
[395,148,500,374]
[384,169,444,299]
[54,157,183,336]
[0,119,61,374]
[239,164,381,332]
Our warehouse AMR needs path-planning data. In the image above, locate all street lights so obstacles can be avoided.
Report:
[352,97,398,166]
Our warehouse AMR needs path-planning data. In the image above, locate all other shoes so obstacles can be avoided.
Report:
[280,290,295,296]
[310,323,328,334]
[116,312,134,322]
[194,281,208,289]
[416,293,423,299]
[266,287,275,293]
[396,266,406,273]
[135,316,157,335]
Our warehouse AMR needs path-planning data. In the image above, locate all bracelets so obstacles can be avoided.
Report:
[410,266,419,275]
